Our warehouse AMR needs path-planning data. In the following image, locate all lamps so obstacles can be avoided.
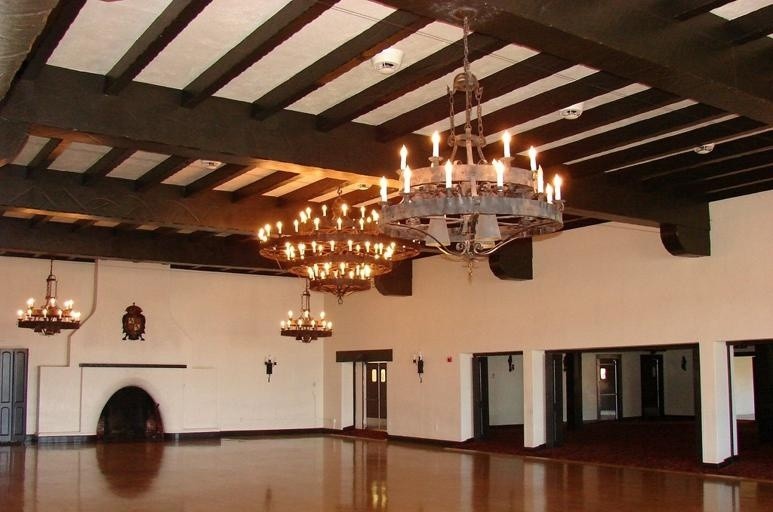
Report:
[257,182,418,343]
[378,7,564,280]
[16,259,80,334]
